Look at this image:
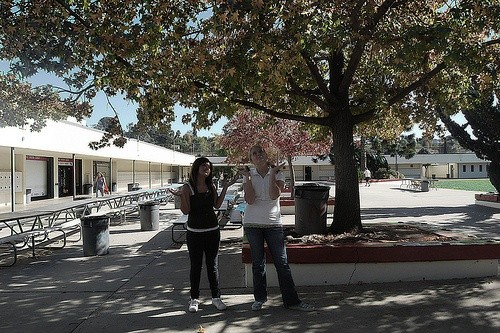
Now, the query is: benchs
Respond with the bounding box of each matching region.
[174,214,188,224]
[230,209,242,223]
[0,183,182,267]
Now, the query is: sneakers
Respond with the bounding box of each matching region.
[252,300,264,310]
[189,299,200,312]
[212,298,226,310]
[289,303,315,311]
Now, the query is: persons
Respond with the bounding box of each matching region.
[363,167,371,187]
[170,157,240,313]
[94,171,106,198]
[237,144,318,314]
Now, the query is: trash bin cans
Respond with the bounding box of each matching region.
[25,188,32,203]
[80,215,110,257]
[83,183,92,195]
[293,185,331,235]
[139,200,160,231]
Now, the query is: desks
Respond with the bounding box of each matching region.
[400,178,439,191]
[71,197,109,217]
[0,210,53,235]
[28,202,82,225]
[99,195,128,211]
[172,183,242,243]
